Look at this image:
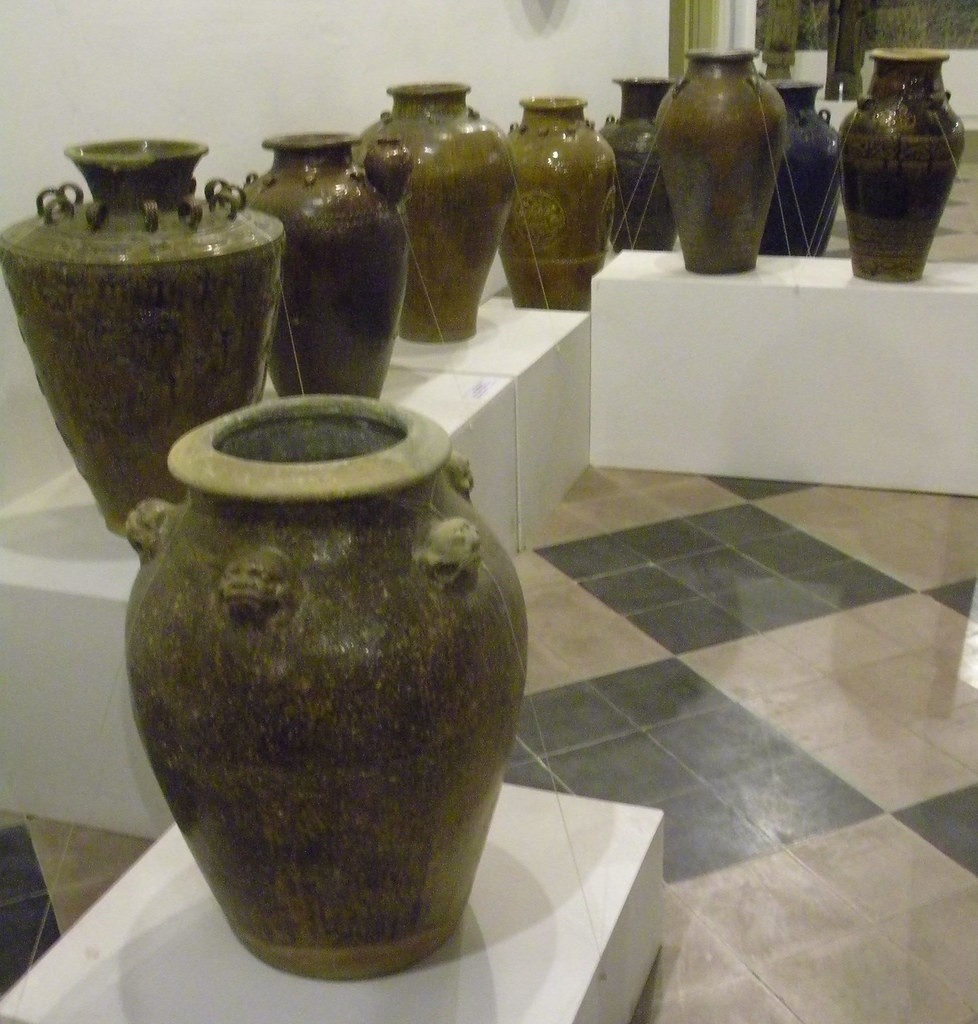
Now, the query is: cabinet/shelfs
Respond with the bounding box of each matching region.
[0,248,978,1023]
